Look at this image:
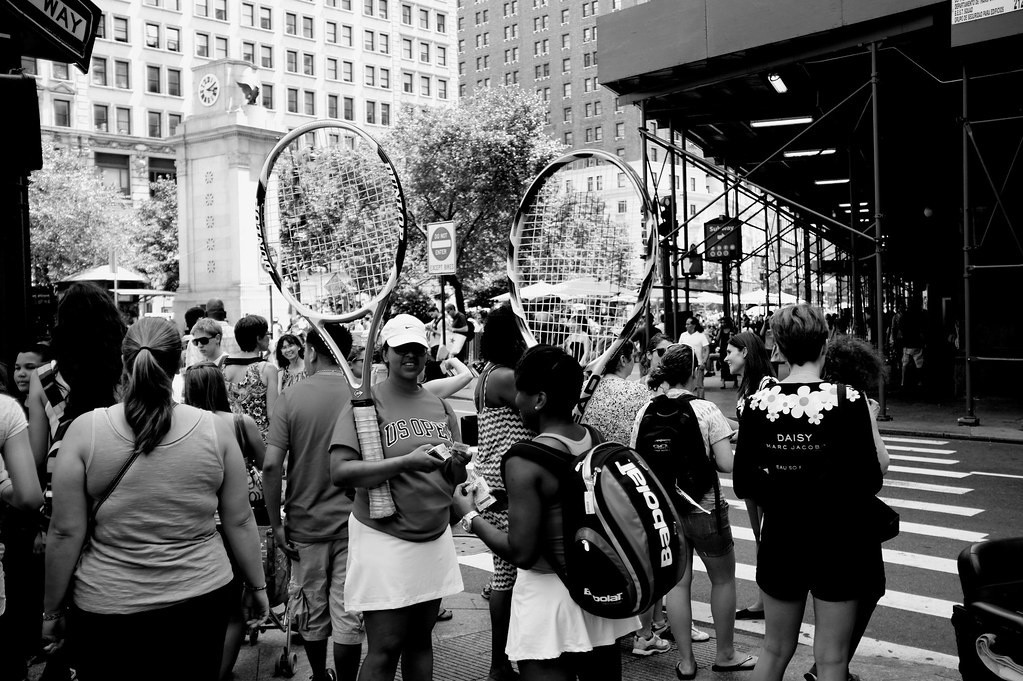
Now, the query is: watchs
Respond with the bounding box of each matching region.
[457,509,480,533]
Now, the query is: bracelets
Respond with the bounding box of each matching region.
[241,582,268,592]
[40,609,69,623]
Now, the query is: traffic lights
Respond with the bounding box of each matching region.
[657,196,676,236]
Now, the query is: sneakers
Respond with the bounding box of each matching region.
[632,620,710,655]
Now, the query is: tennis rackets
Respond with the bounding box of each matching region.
[502,146,661,425]
[252,117,412,521]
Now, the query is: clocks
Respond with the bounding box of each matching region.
[197,73,221,108]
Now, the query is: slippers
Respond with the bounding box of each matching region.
[675,659,697,680]
[712,655,758,672]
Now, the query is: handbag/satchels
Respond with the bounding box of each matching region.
[234,413,263,506]
[440,328,467,354]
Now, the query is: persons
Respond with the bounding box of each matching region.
[626,345,763,678]
[11,344,47,453]
[451,343,634,680]
[632,361,714,643]
[24,280,137,680]
[259,322,374,681]
[723,331,779,624]
[732,306,888,681]
[36,316,272,680]
[174,296,951,423]
[171,360,270,678]
[474,305,546,680]
[571,334,689,656]
[327,314,467,679]
[379,351,475,622]
[0,393,50,681]
[800,327,884,681]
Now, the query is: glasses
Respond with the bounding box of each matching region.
[447,310,451,313]
[393,345,426,356]
[666,344,694,379]
[191,337,216,346]
[265,332,273,339]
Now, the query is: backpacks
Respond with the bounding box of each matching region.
[465,321,475,342]
[500,421,688,618]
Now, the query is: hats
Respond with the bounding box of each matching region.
[380,313,429,349]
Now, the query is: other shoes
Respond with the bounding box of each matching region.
[735,607,764,619]
[804,665,860,681]
[437,608,452,620]
[309,668,336,681]
